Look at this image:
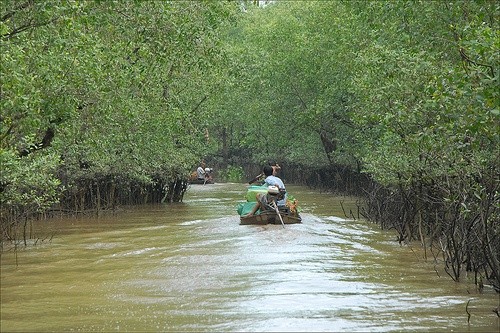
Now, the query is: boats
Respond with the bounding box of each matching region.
[239,202,303,226]
[188,172,215,185]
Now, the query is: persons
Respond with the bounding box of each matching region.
[197,164,205,180]
[247,191,275,217]
[263,165,286,207]
